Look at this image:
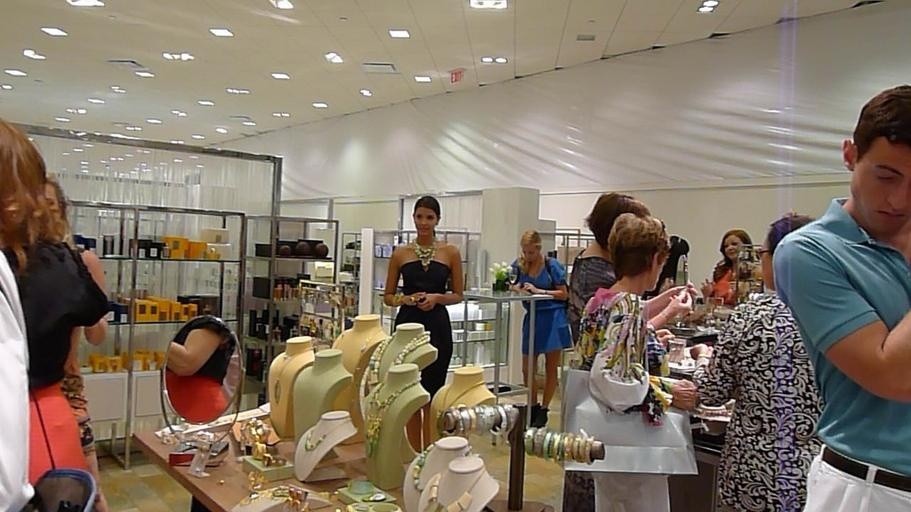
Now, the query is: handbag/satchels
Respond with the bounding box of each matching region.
[27,468,96,511]
[563,368,698,475]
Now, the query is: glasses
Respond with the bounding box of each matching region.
[757,249,770,259]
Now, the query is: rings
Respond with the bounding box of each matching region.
[410,296,417,302]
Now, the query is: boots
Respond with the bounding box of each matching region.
[531,402,548,428]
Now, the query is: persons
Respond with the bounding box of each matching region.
[0,119,112,511]
[687,213,833,511]
[775,83,911,511]
[701,227,756,304]
[383,195,466,408]
[504,229,574,430]
[0,250,39,512]
[164,315,237,425]
[42,176,109,511]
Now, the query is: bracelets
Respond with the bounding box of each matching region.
[660,311,672,323]
[437,403,595,465]
[393,292,405,307]
[545,289,548,294]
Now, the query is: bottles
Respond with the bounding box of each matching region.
[410,295,426,303]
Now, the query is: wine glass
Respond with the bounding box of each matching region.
[506,268,519,295]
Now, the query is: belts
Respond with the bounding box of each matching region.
[820,448,911,493]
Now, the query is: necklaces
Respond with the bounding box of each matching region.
[422,467,491,511]
[413,438,473,493]
[367,330,433,386]
[304,418,352,451]
[364,376,423,457]
[410,238,439,272]
[435,380,488,412]
[271,346,315,405]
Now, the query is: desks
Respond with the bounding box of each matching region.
[131,420,404,511]
